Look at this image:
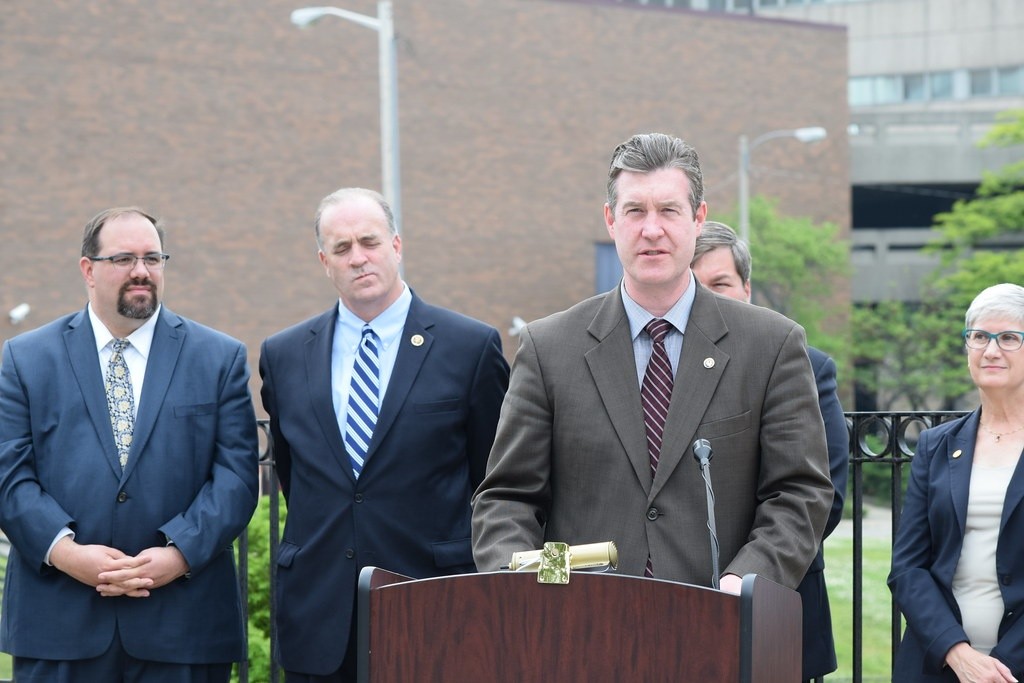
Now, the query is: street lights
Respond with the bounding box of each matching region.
[291,0,403,285]
[739,126,828,302]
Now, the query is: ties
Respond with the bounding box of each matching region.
[345,324,380,480]
[641,319,674,482]
[106,337,136,475]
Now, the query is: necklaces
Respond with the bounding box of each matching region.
[979,418,1024,442]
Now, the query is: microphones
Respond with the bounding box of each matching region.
[693,438,721,590]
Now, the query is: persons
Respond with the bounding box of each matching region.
[258,188,511,683]
[886,283,1024,683]
[690,221,851,683]
[0,206,259,683]
[470,133,835,594]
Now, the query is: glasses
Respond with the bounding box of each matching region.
[91,254,169,271]
[962,328,1024,351]
